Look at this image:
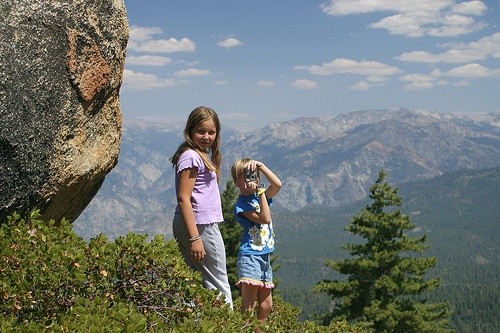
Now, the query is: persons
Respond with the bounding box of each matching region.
[168,106,235,314]
[231,157,282,333]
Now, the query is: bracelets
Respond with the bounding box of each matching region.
[257,188,266,197]
[189,235,202,241]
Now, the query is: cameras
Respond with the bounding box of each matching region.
[245,168,259,184]
